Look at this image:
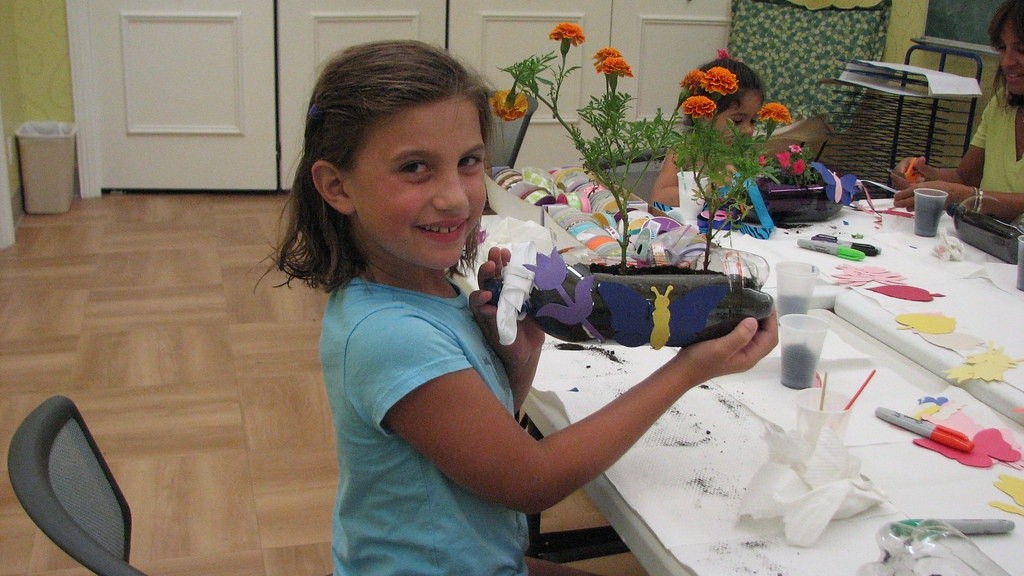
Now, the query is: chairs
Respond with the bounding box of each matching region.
[6,393,154,576]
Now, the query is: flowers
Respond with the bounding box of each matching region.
[756,143,822,187]
[484,18,792,273]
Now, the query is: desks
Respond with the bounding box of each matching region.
[444,192,1023,576]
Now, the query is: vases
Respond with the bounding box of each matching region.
[486,254,776,353]
[719,166,863,229]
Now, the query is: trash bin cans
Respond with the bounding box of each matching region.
[15,120,80,216]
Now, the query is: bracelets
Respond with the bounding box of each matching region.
[972,185,982,214]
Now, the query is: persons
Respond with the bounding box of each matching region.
[889,0,1024,229]
[650,57,767,208]
[257,38,778,576]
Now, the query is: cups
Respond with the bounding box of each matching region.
[1016,235,1024,291]
[914,188,949,238]
[795,387,853,450]
[677,171,708,221]
[779,314,829,390]
[774,260,819,325]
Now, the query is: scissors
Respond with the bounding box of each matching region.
[885,157,925,188]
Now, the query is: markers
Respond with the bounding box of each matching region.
[874,406,976,452]
[797,237,866,262]
[811,233,878,257]
[892,518,1016,536]
